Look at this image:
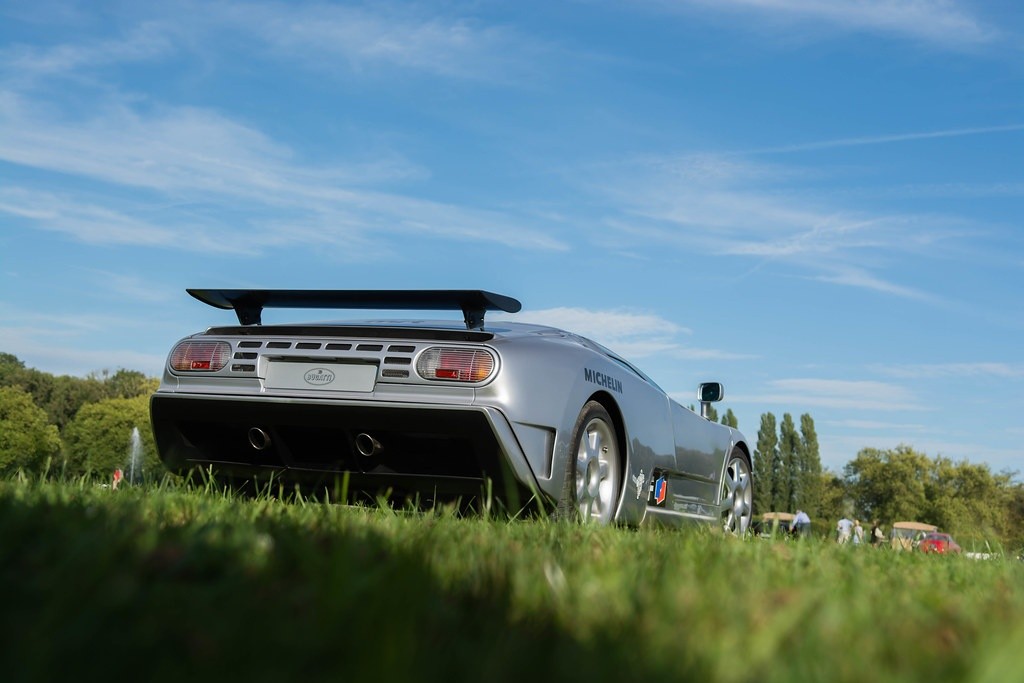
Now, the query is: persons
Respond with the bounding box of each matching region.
[789,509,811,534]
[869,520,877,544]
[112,467,123,490]
[836,515,854,543]
[874,522,887,544]
[853,520,863,542]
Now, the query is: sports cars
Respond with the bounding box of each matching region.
[150,287,756,535]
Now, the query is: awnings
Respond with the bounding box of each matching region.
[762,511,793,520]
[890,520,937,549]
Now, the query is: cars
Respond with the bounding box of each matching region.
[918,532,961,555]
